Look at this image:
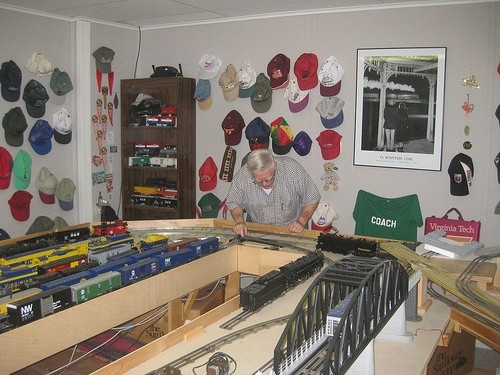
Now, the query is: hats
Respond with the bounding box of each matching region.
[447,152,472,196]
[198,156,218,191]
[1,51,76,240]
[217,50,346,160]
[198,193,222,219]
[311,200,337,231]
[93,45,115,72]
[196,51,223,80]
[193,79,213,110]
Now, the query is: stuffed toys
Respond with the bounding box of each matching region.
[321,163,341,191]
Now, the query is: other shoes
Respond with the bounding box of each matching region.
[386,147,395,152]
[397,147,404,152]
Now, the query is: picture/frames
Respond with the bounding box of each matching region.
[353,46,447,171]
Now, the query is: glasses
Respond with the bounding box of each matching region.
[251,167,276,184]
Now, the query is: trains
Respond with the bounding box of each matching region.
[326,283,381,337]
[240,249,325,311]
[1,219,219,325]
[316,233,377,258]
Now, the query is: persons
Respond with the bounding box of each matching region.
[224,148,321,237]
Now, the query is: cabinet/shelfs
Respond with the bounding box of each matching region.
[120,77,195,220]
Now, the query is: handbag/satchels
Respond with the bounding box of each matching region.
[424,207,480,244]
